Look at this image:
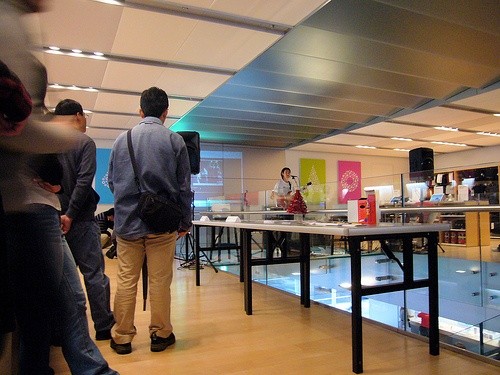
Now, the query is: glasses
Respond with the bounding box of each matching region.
[85,114,89,118]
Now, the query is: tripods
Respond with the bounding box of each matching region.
[176,232,218,274]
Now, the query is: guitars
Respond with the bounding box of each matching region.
[277,182,312,207]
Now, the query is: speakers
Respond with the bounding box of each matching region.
[177,131,200,174]
[409,148,434,183]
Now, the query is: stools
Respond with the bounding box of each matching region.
[210,204,239,261]
[327,216,347,254]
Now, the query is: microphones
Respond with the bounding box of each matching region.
[290,175,297,177]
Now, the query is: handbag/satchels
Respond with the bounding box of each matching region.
[137,190,185,234]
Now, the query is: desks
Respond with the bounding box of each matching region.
[192,220,452,374]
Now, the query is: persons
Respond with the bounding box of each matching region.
[0,50,116,375]
[472,183,485,197]
[423,190,432,200]
[270,168,294,207]
[107,86,193,354]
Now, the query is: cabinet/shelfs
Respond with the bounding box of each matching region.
[384,201,490,247]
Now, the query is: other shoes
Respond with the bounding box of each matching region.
[150,333,176,352]
[95,326,137,340]
[109,338,133,355]
